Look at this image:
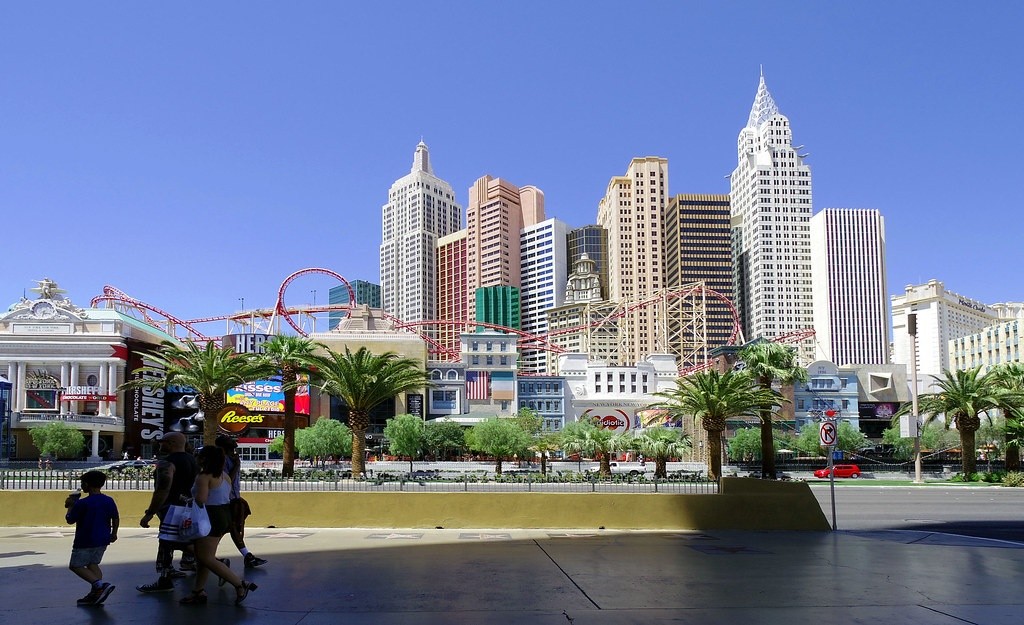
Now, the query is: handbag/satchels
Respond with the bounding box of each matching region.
[230,496,251,531]
[157,496,190,542]
[178,499,212,540]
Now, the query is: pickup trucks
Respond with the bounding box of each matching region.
[590,462,649,478]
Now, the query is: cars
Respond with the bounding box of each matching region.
[814,464,861,479]
[106,459,157,477]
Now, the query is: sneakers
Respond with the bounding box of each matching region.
[136,578,174,593]
[217,558,230,586]
[77,592,94,605]
[90,582,116,606]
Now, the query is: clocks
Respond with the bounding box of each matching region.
[31,302,54,318]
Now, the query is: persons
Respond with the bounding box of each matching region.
[64,470,119,605]
[136,432,267,592]
[179,446,257,604]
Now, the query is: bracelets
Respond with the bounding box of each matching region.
[145,510,151,516]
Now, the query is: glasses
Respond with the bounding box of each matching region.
[158,439,175,444]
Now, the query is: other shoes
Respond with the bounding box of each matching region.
[168,569,188,578]
[242,552,268,568]
[182,562,197,572]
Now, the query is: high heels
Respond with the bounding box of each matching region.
[179,588,207,605]
[234,580,258,604]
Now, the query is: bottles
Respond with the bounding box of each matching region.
[64,488,82,508]
